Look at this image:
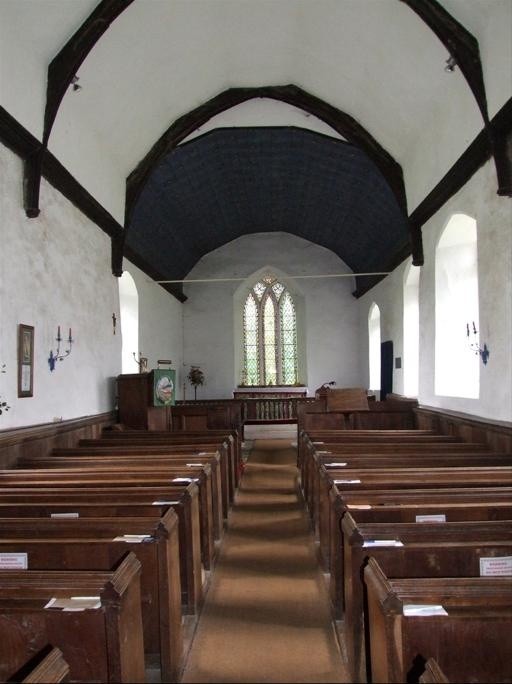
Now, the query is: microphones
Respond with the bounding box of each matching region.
[322,382,335,387]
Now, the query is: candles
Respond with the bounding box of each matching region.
[467,323,469,333]
[472,321,476,331]
[58,326,60,338]
[69,328,71,337]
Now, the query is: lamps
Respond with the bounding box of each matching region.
[445,57,457,72]
[70,74,82,93]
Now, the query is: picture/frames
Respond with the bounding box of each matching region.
[18,324,34,398]
